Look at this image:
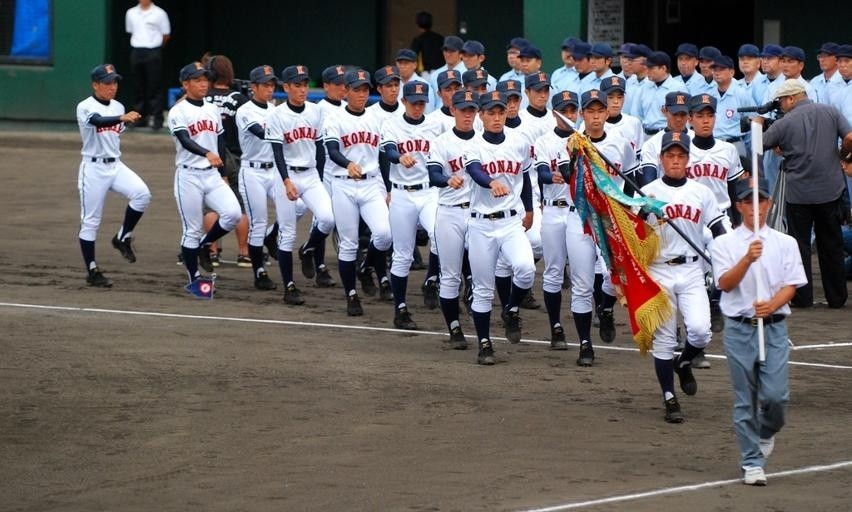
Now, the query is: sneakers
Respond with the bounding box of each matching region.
[448,319,467,349]
[597,305,616,343]
[577,339,594,367]
[86,266,113,288]
[551,323,568,350]
[237,223,424,317]
[111,232,136,263]
[477,338,495,365]
[394,303,417,331]
[707,288,724,332]
[198,235,220,272]
[672,326,711,369]
[673,354,697,396]
[421,274,439,310]
[759,434,774,458]
[741,462,767,486]
[501,293,541,344]
[664,391,683,423]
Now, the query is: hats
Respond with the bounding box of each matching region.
[689,94,718,113]
[91,63,122,83]
[736,176,770,200]
[617,43,852,70]
[661,131,690,153]
[437,35,626,111]
[771,79,806,104]
[665,90,691,115]
[178,49,430,103]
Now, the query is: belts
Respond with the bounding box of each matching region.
[666,255,699,265]
[290,166,309,174]
[544,199,576,212]
[394,182,435,192]
[334,173,376,181]
[250,162,274,170]
[184,165,213,171]
[91,157,115,163]
[453,201,470,209]
[727,313,785,327]
[471,209,517,221]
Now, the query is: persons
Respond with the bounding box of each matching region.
[628,132,728,424]
[125,0,173,130]
[76,63,152,289]
[379,36,546,365]
[706,174,808,489]
[410,10,447,77]
[269,62,402,315]
[167,56,308,298]
[534,36,852,367]
